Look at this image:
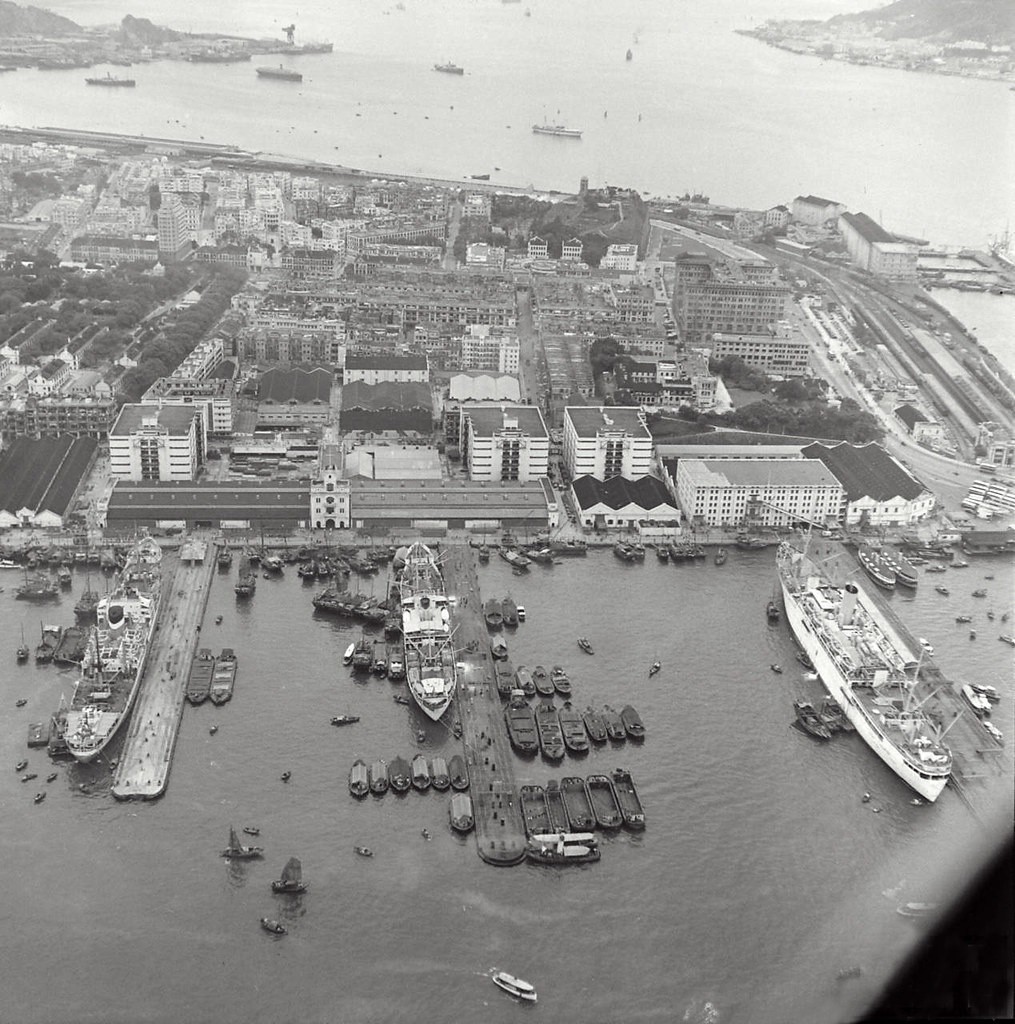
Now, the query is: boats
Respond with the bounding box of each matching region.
[1,517,1015,934]
[773,523,967,801]
[389,540,459,722]
[55,519,165,765]
[492,970,539,1004]
